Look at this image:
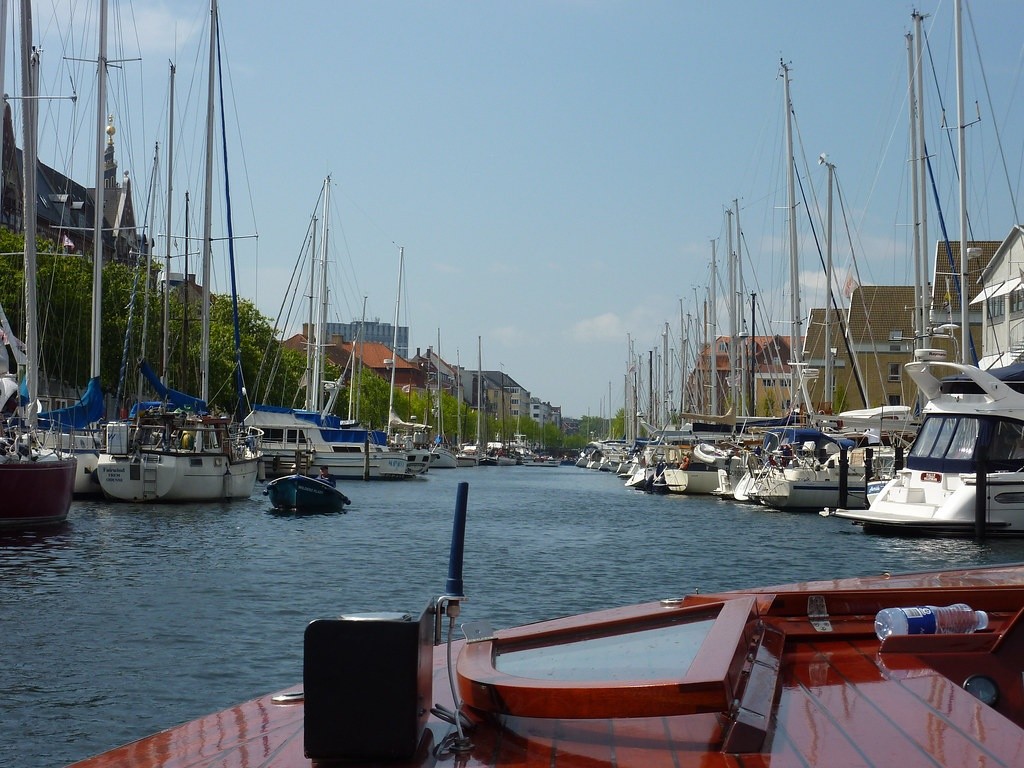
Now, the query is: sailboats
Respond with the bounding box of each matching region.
[3,1,1023,543]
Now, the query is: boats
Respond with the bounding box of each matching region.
[59,480,1024,768]
[264,477,353,516]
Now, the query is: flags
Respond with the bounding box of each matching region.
[841,264,859,299]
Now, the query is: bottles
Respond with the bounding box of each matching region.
[874,603,990,640]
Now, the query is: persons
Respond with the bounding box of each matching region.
[316,465,336,489]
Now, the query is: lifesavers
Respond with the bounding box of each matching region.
[681,456,690,469]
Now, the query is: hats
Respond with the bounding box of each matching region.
[320,465,328,469]
